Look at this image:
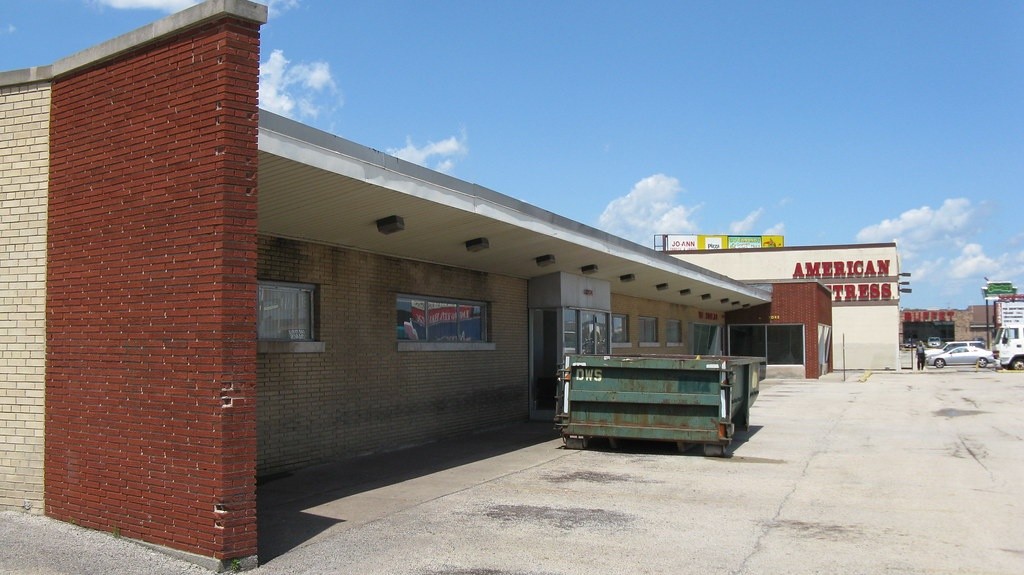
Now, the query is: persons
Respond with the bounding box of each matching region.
[916,341,926,370]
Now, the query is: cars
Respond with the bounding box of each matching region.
[928,337,941,348]
[926,346,994,368]
[904,341,916,348]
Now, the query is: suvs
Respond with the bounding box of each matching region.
[922,340,985,365]
[992,323,1024,370]
